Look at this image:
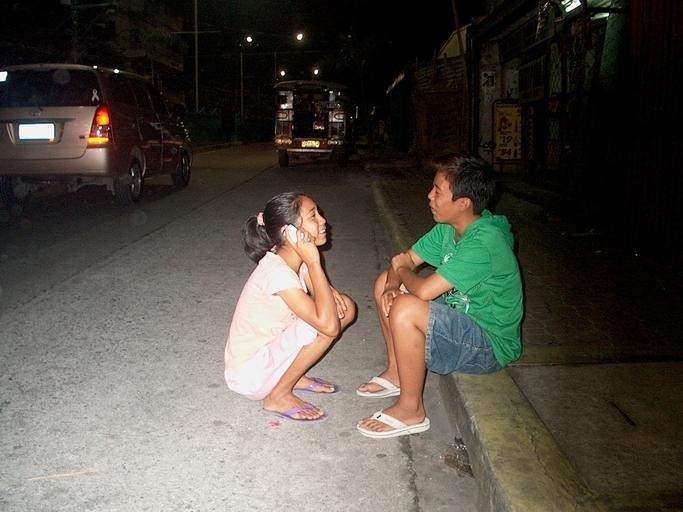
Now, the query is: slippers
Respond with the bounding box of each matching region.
[356,407,431,438]
[262,395,330,424]
[356,377,401,398]
[293,377,342,396]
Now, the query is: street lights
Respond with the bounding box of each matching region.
[239,32,254,118]
[271,25,323,81]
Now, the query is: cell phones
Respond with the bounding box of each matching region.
[282,223,310,244]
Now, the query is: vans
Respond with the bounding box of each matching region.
[0,61,196,204]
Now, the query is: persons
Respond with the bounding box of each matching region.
[355,152,523,439]
[221,193,359,423]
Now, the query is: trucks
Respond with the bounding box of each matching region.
[268,80,354,170]
